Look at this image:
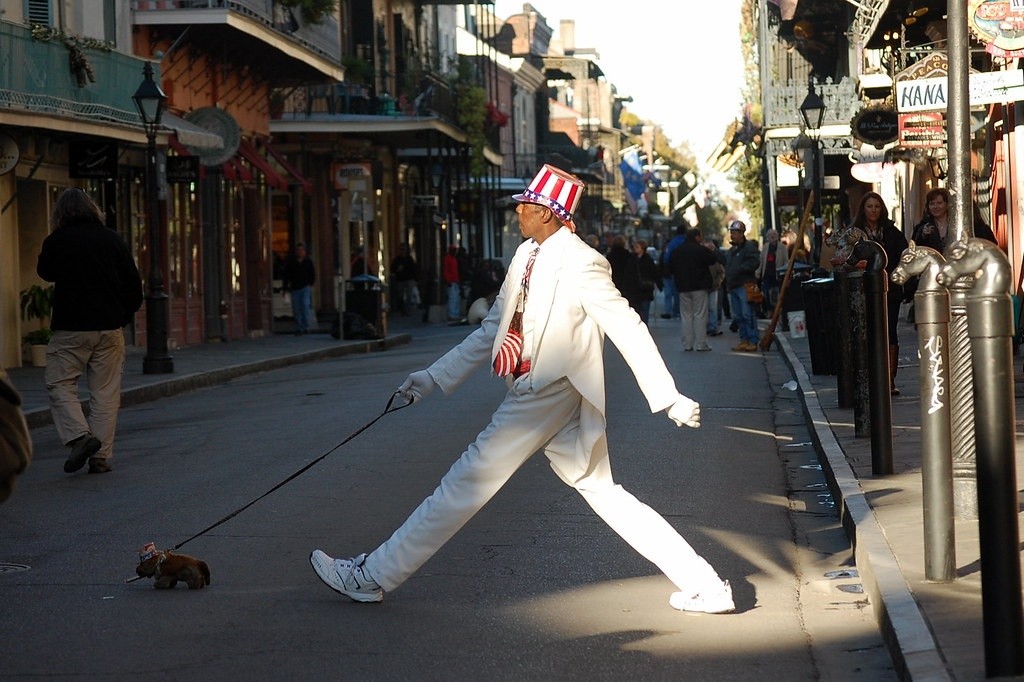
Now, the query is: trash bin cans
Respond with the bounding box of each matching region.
[344,274,384,340]
[776,261,812,331]
[800,277,839,376]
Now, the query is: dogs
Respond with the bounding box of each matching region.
[136,542,210,589]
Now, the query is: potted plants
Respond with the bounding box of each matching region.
[18,284,55,368]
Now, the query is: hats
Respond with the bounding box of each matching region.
[449,243,460,250]
[511,164,586,233]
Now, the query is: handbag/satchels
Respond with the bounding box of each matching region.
[746,283,763,304]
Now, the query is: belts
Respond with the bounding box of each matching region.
[511,360,530,379]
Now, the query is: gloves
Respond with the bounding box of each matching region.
[396,370,437,408]
[666,393,701,428]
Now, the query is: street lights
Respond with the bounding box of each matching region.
[131,60,178,374]
[798,76,828,278]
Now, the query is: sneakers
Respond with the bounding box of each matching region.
[669,579,735,614]
[310,550,383,602]
[88,458,112,473]
[64,435,102,473]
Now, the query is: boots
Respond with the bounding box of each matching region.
[889,344,900,395]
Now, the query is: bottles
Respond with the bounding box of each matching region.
[745,283,761,302]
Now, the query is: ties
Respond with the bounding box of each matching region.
[492,247,541,378]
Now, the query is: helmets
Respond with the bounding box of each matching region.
[728,221,746,231]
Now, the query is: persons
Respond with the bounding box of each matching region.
[578,225,789,355]
[908,189,999,328]
[443,245,481,321]
[390,242,421,316]
[280,242,316,334]
[0,364,34,507]
[35,189,145,474]
[309,165,735,615]
[846,193,912,395]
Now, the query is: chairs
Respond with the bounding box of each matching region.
[307,79,375,115]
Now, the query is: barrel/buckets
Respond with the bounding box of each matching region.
[787,311,806,338]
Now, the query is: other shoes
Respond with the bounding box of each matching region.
[731,341,757,351]
[712,330,722,336]
[697,344,712,351]
[685,344,693,351]
[729,324,737,332]
[661,313,670,318]
